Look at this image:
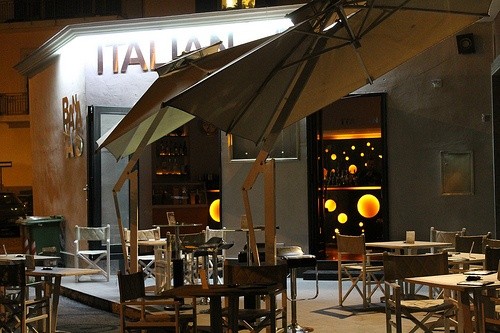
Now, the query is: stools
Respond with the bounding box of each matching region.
[239,242,265,324]
[278,244,319,333]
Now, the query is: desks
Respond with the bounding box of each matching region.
[404,274,500,333]
[25,267,101,333]
[161,283,287,333]
[209,226,279,265]
[0,253,61,300]
[153,224,203,259]
[365,240,452,300]
[428,252,485,270]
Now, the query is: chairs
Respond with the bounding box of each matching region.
[117,227,288,333]
[335,226,500,333]
[0,222,112,333]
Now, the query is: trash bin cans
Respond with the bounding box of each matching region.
[16,214,65,267]
